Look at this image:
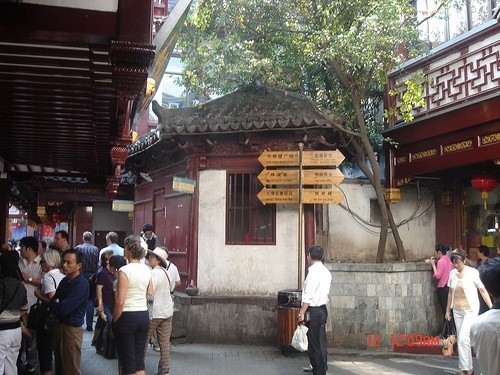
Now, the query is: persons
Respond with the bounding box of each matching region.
[0,230,129,375]
[297,246,332,375]
[146,246,177,375]
[430,242,500,375]
[112,235,154,375]
[0,252,29,375]
[140,223,160,261]
[48,248,91,375]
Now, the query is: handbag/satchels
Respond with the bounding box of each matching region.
[291,321,309,352]
[442,339,452,356]
[26,298,52,331]
[103,325,119,359]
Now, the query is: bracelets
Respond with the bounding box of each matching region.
[28,278,33,283]
[298,313,304,317]
[100,309,104,312]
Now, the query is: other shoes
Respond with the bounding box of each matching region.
[151,346,160,351]
[303,365,328,372]
[25,363,37,375]
[86,327,93,331]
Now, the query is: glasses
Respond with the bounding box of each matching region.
[40,260,45,264]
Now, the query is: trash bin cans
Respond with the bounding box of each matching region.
[276,289,306,356]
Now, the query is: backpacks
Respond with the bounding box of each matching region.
[88,271,95,300]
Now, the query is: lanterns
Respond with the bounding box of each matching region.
[471,173,497,211]
[17,221,22,228]
[53,210,66,224]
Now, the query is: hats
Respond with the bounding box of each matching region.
[147,247,170,269]
